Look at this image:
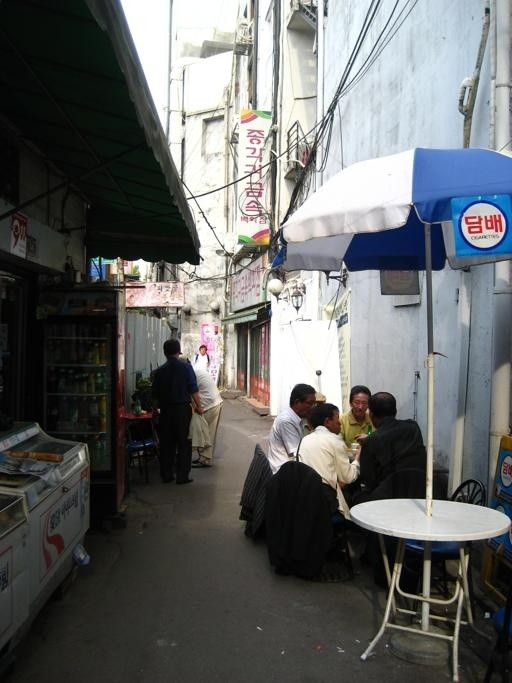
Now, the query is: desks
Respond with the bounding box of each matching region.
[348,497,512,683]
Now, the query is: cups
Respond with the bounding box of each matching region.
[351,442,361,448]
[73,270,81,282]
[352,449,357,458]
[88,275,98,282]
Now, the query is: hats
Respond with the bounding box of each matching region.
[163,339,183,355]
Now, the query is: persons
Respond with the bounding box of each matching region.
[148,338,224,482]
[267,382,428,574]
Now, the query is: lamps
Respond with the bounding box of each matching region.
[290,279,307,312]
[266,277,289,304]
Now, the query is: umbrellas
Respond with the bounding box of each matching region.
[274,148,512,517]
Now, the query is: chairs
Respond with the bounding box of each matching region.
[125,420,156,483]
[242,443,274,547]
[403,478,487,625]
[283,461,357,582]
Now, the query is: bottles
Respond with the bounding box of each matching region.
[70,435,107,469]
[65,255,74,281]
[51,396,106,432]
[367,423,373,435]
[50,367,108,392]
[134,394,142,414]
[48,339,107,364]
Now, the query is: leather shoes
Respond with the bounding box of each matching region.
[163,458,211,484]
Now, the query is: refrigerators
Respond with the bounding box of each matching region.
[40,282,128,514]
